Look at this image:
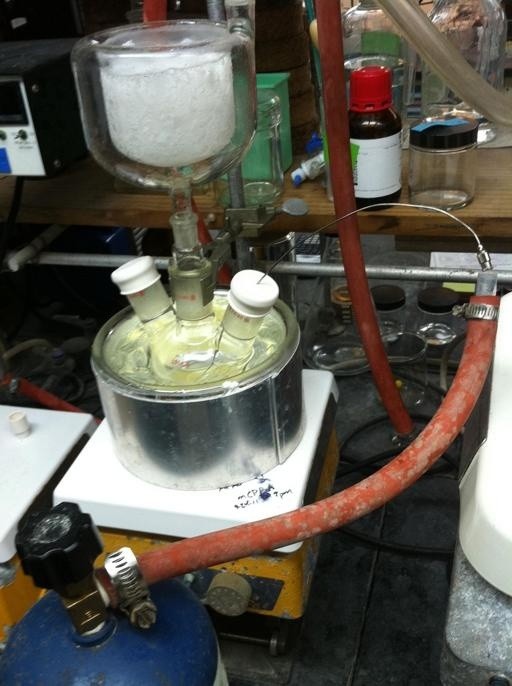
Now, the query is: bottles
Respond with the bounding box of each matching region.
[370,285,407,342]
[414,286,462,345]
[407,117,477,213]
[347,65,405,212]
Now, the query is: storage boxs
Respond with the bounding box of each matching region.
[219,70,293,180]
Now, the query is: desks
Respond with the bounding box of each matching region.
[0,144,511,291]
